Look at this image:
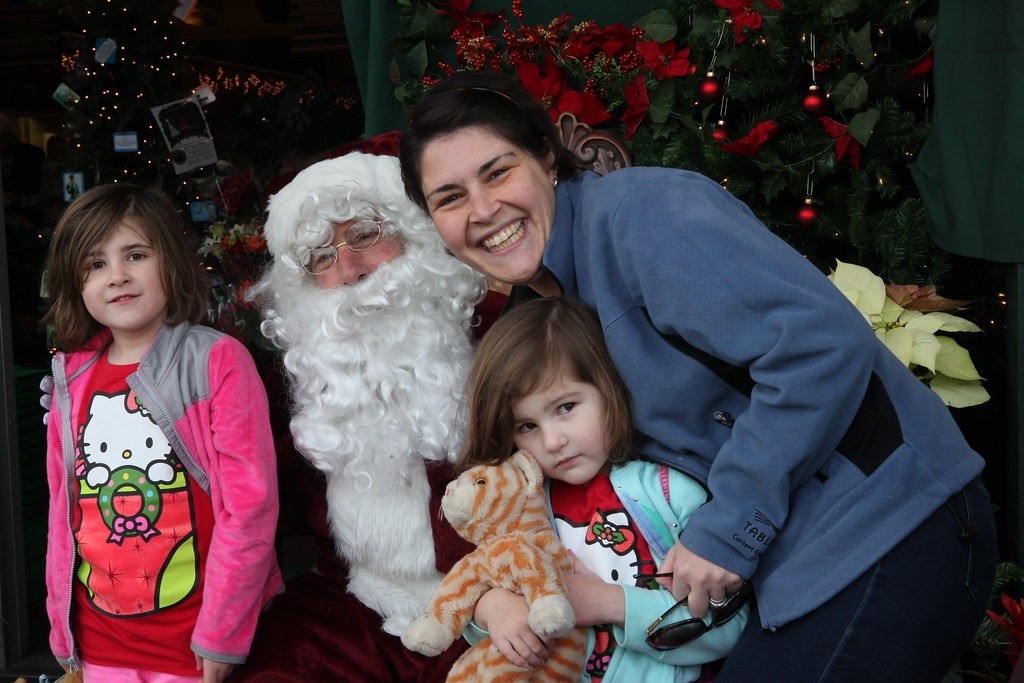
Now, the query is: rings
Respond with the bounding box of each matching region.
[710,598,725,607]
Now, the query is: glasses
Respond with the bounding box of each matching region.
[633,570,750,652]
[301,217,390,274]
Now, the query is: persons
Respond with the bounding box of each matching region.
[393,72,1004,683]
[456,296,748,683]
[39,131,508,683]
[37,181,283,683]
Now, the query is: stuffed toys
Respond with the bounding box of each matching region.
[401,446,589,683]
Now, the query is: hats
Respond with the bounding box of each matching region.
[265,132,412,261]
